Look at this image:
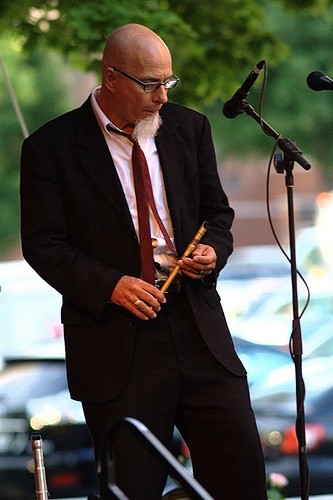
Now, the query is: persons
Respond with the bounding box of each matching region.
[21,24,268,500]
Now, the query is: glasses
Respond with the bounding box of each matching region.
[111,66,179,93]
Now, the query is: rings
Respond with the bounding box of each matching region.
[135,300,141,304]
[200,270,204,275]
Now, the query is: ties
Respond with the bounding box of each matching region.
[107,125,174,286]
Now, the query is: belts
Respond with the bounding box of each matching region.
[155,279,183,294]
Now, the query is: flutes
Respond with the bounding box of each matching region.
[143,219,210,318]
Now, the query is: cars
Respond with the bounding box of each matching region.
[0,244,333,500]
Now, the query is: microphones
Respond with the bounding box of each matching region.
[306,71,333,91]
[222,60,265,118]
